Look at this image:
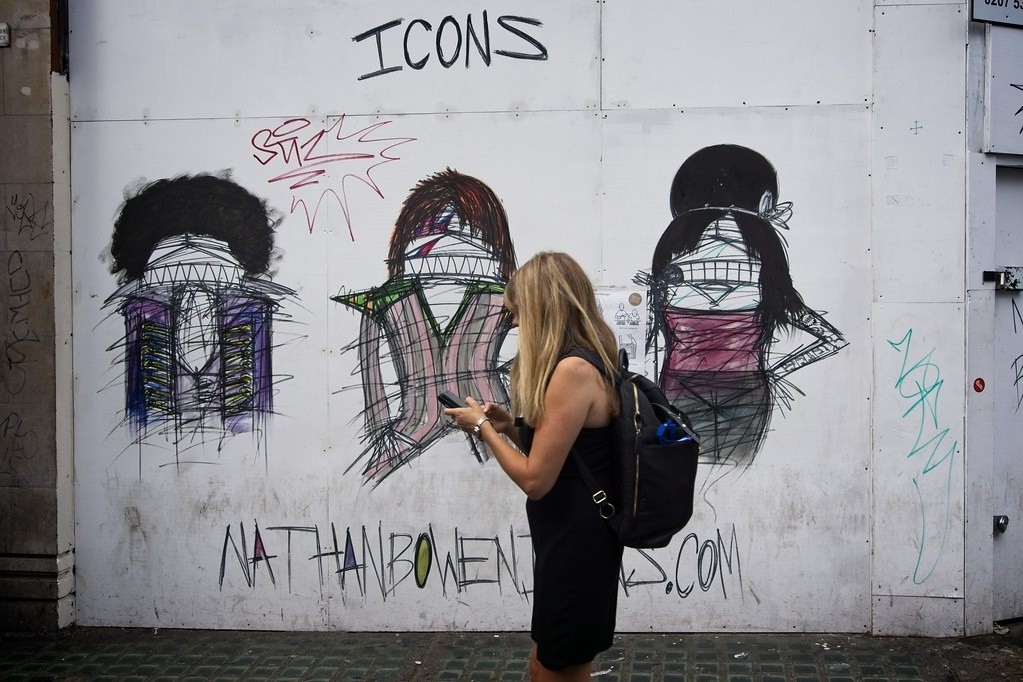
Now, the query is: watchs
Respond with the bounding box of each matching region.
[473,418,493,441]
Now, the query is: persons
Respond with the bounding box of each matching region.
[446,252,621,682]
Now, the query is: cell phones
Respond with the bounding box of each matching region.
[438,391,469,410]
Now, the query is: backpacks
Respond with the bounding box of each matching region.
[542,345,704,550]
